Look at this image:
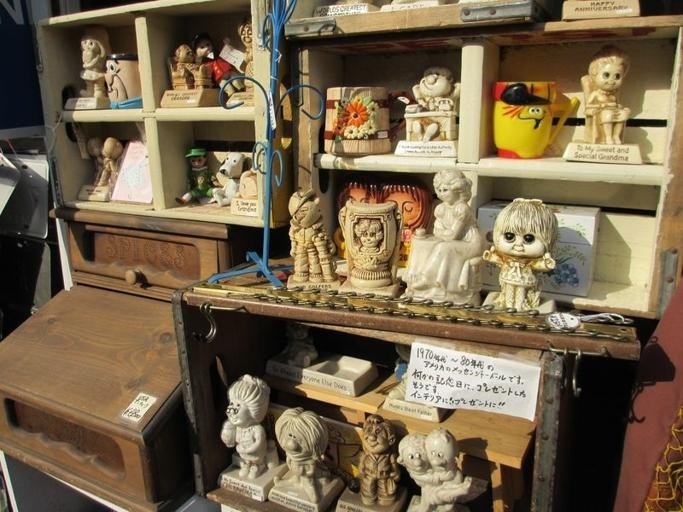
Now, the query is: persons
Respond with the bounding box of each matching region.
[76,30,109,100]
[267,406,343,511]
[190,31,244,99]
[395,429,474,511]
[216,373,288,502]
[422,428,464,511]
[335,413,410,511]
[581,43,633,148]
[174,146,213,203]
[234,16,254,94]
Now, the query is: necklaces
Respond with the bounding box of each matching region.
[597,89,617,96]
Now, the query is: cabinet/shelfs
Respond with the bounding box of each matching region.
[284,15,683,319]
[0,208,257,512]
[36,0,333,230]
[172,256,641,512]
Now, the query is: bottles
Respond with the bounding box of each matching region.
[104,51,142,110]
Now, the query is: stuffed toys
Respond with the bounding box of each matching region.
[212,151,250,206]
[96,137,123,187]
[87,135,103,185]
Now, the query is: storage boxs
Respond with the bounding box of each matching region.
[477,200,601,297]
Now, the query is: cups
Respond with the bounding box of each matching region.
[489,80,580,161]
